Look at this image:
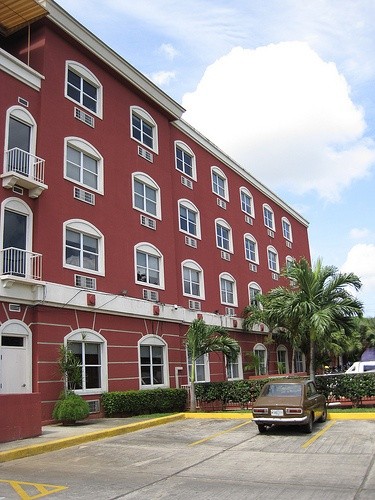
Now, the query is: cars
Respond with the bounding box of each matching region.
[251,379,327,433]
[345,361,375,374]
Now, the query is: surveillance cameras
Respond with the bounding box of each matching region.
[174,304,178,310]
